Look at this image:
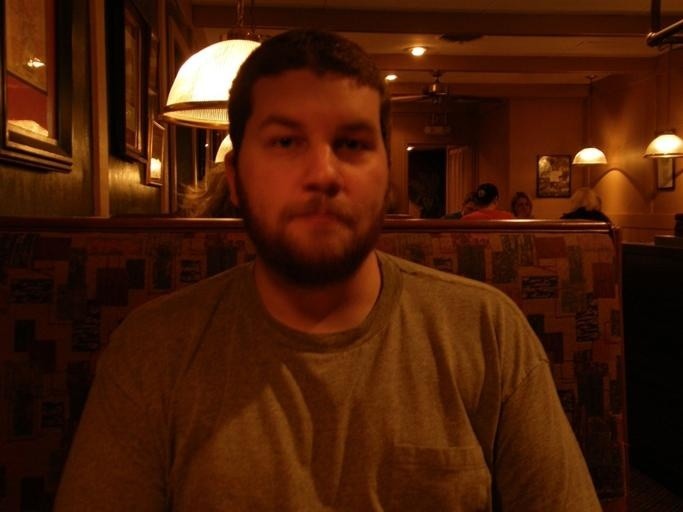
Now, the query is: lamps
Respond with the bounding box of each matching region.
[641,128,683,160]
[160,38,263,131]
[571,147,608,166]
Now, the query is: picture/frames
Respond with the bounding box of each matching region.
[107,3,166,186]
[0,0,76,172]
[654,128,676,192]
[537,153,572,199]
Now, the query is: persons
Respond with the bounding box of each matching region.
[511,191,534,218]
[459,181,514,221]
[440,191,478,220]
[559,188,612,224]
[188,163,240,218]
[51,29,602,511]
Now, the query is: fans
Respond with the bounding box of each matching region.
[388,67,504,106]
